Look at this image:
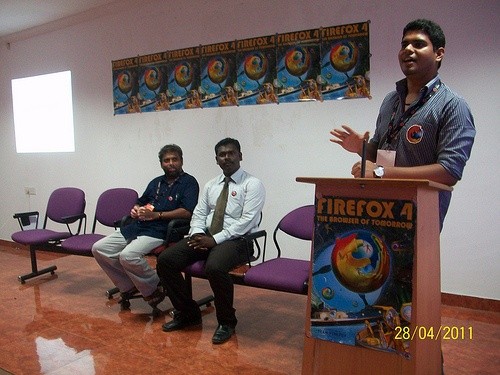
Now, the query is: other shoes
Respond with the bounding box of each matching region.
[148,287,166,305]
[118,286,144,302]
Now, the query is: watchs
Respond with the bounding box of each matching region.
[373,165,384,178]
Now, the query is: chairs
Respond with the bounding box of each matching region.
[11,187,315,318]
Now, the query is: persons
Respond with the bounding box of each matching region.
[155,137,264,344]
[329,18,476,375]
[91,143,200,306]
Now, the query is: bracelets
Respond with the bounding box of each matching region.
[159,211,163,218]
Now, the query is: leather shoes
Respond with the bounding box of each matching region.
[212,324,234,345]
[163,307,202,332]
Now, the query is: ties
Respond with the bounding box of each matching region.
[209,176,232,236]
